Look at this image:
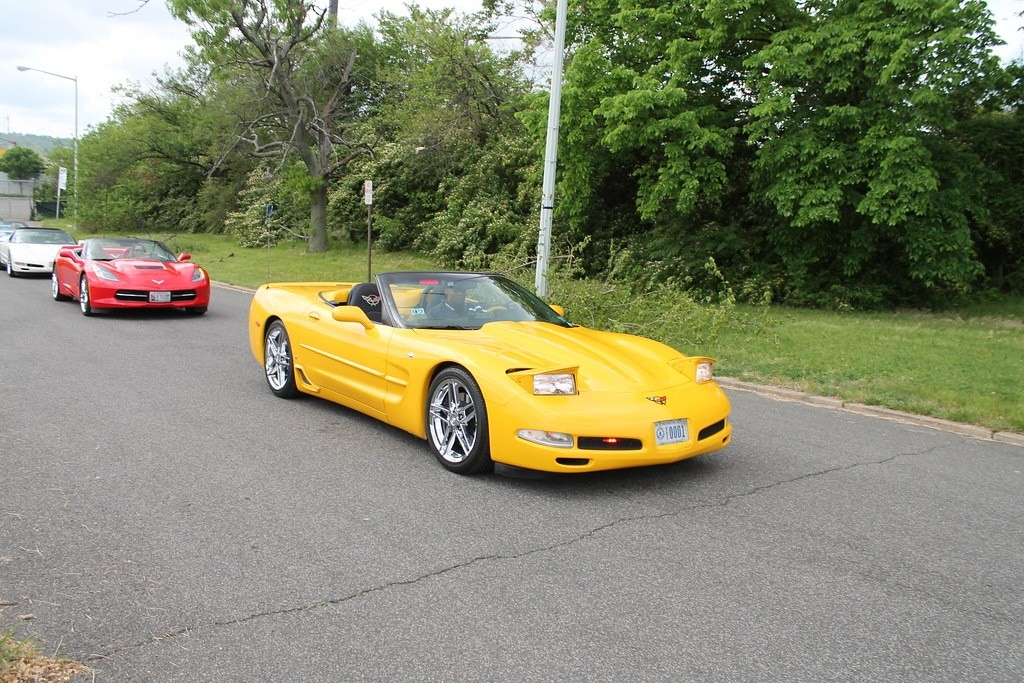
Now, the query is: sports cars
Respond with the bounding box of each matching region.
[49,233,211,318]
[247,271,731,476]
[1,227,79,278]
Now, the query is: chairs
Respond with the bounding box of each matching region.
[78,245,107,259]
[346,282,383,323]
[124,247,134,258]
[46,233,61,244]
[20,235,33,243]
[420,284,448,319]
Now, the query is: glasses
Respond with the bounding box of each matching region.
[135,249,142,252]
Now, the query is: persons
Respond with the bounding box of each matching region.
[128,243,151,258]
[47,232,58,241]
[427,286,494,317]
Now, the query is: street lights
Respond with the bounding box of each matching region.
[18,65,79,234]
